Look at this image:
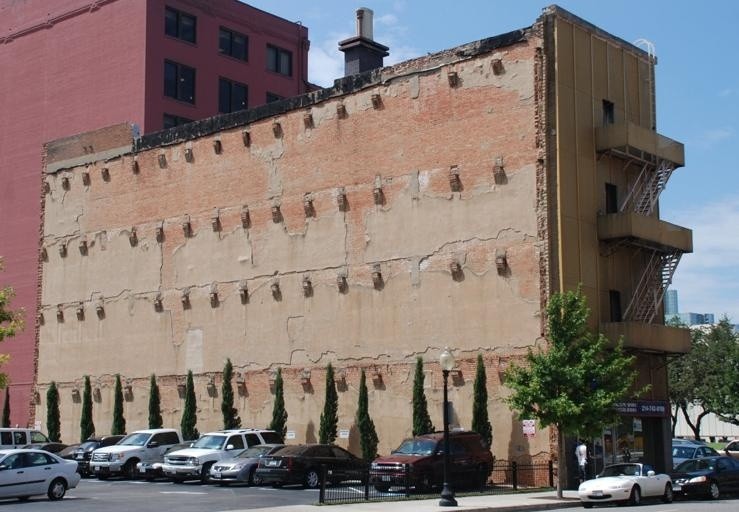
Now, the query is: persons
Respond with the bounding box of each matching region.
[575,438,589,483]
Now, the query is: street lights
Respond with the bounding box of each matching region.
[437,346,460,506]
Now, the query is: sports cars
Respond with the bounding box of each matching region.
[578,462,673,507]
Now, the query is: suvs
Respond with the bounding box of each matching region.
[162,425,285,485]
[369,429,494,497]
[89,427,183,480]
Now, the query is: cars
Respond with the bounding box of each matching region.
[209,444,287,487]
[1,448,82,502]
[607,447,646,465]
[671,437,738,501]
[255,444,364,489]
[135,440,196,482]
[22,433,128,479]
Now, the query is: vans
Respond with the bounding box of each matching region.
[0,427,51,459]
